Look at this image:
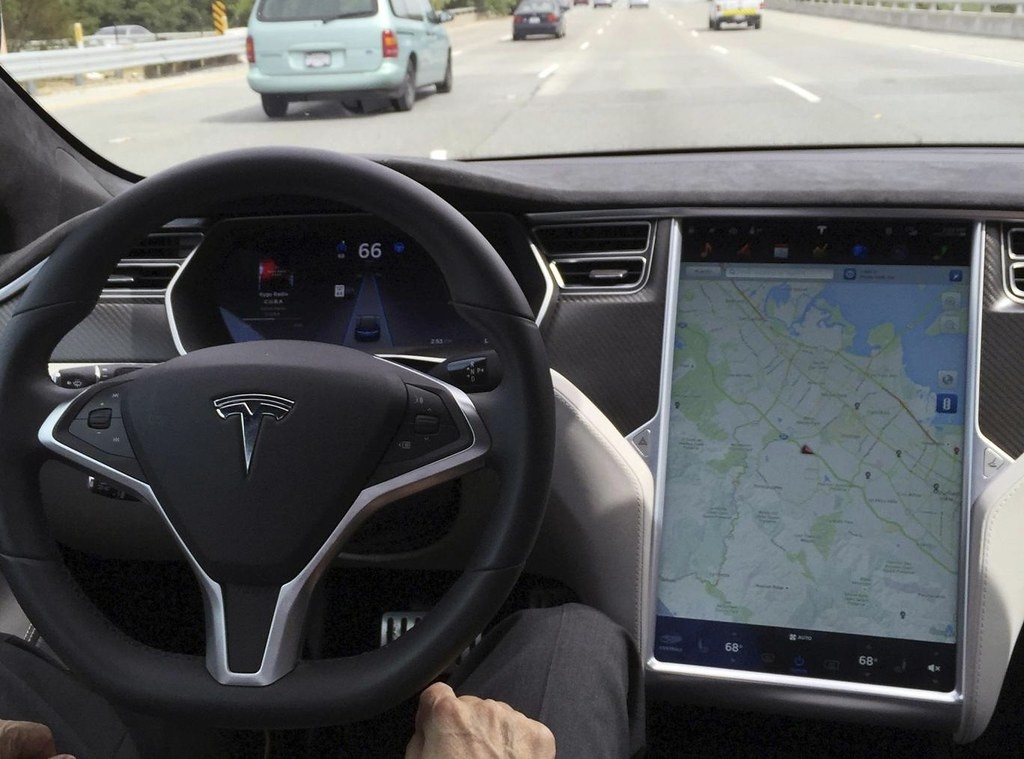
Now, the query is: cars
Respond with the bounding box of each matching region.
[594,0,612,7]
[708,0,763,31]
[511,0,569,41]
[246,0,454,118]
[630,0,649,9]
[574,0,588,6]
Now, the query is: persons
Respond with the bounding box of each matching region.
[0,602,648,759]
